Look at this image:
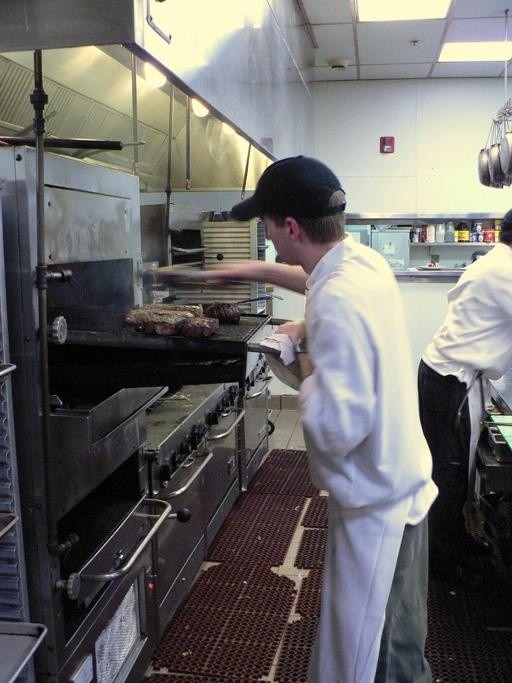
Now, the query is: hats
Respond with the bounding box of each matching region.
[231,155,346,221]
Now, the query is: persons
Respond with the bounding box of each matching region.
[416,206,512,586]
[153,149,440,683]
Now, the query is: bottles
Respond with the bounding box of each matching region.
[412,221,471,243]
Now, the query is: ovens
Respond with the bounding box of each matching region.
[2,183,276,527]
[22,319,309,682]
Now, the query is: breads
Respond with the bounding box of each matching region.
[137,303,204,317]
[183,316,220,339]
[205,301,240,325]
[124,308,193,336]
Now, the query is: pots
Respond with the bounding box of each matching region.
[477,113,512,189]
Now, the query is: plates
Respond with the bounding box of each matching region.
[421,264,466,270]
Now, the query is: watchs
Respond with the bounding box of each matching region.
[291,336,310,353]
